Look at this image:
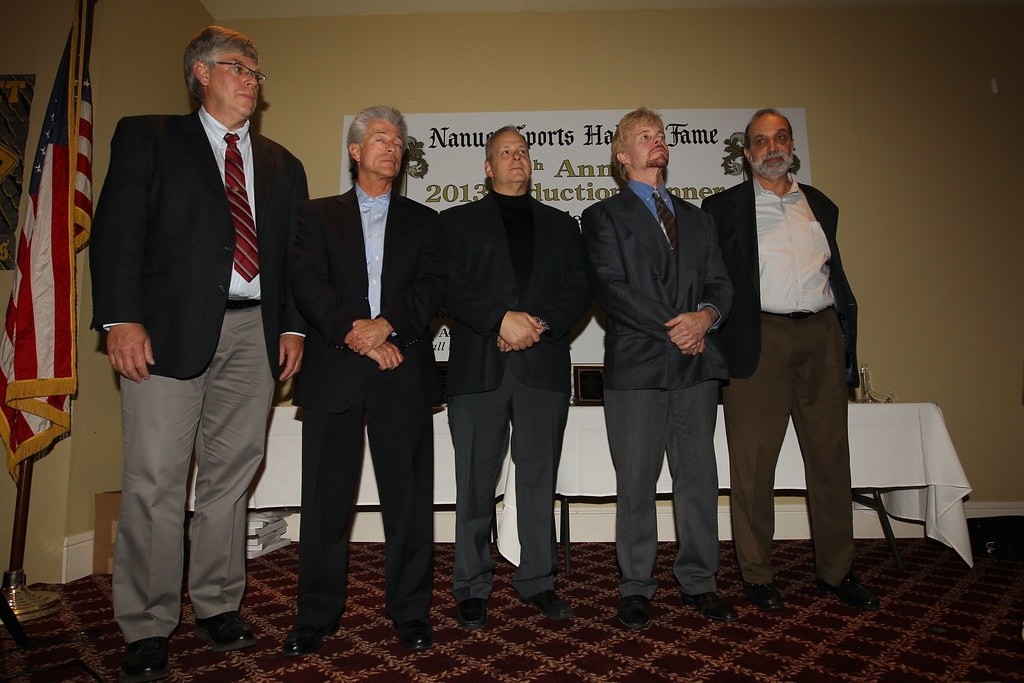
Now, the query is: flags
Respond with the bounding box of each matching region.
[0,0,99,483]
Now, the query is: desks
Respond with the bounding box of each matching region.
[183,392,975,579]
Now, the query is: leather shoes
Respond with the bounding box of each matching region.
[819,572,877,607]
[617,594,652,629]
[398,616,434,649]
[195,611,258,652]
[119,636,171,683]
[679,589,739,620]
[457,598,488,630]
[283,623,336,655]
[529,589,573,620]
[745,580,785,614]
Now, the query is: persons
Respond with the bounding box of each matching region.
[700,109,881,611]
[283,105,446,654]
[437,125,594,627]
[90,26,309,683]
[581,107,736,627]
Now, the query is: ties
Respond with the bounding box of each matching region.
[652,189,678,266]
[223,132,260,283]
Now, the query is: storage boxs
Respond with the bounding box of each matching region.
[91,489,123,574]
[244,518,291,559]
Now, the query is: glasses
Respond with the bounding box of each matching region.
[215,61,266,85]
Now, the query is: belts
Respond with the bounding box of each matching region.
[768,305,831,319]
[225,299,262,312]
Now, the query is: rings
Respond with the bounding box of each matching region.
[355,346,361,351]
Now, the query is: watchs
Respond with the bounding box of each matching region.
[533,316,551,332]
